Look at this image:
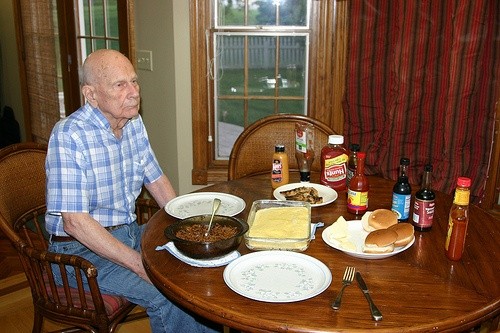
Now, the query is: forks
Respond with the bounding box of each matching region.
[331,266,356,310]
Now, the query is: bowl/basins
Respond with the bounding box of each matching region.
[163,213,249,258]
[245,199,312,251]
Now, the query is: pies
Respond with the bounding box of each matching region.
[280,186,322,204]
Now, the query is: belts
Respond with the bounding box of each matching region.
[49,224,116,242]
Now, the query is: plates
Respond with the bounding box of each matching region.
[223,250,333,304]
[273,182,338,208]
[322,220,416,259]
[164,192,247,221]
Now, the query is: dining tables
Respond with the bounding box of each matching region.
[141,169,500,333]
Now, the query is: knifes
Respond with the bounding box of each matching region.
[355,271,383,319]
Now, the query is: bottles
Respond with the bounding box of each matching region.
[391,157,412,223]
[270,144,289,189]
[319,135,352,191]
[346,145,363,185]
[412,164,436,232]
[293,121,316,181]
[347,151,369,215]
[445,177,471,261]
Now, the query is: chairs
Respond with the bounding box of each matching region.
[0,139,165,332]
[227,112,348,186]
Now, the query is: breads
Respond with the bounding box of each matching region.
[327,209,414,254]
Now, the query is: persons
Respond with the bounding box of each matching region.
[45,49,223,333]
